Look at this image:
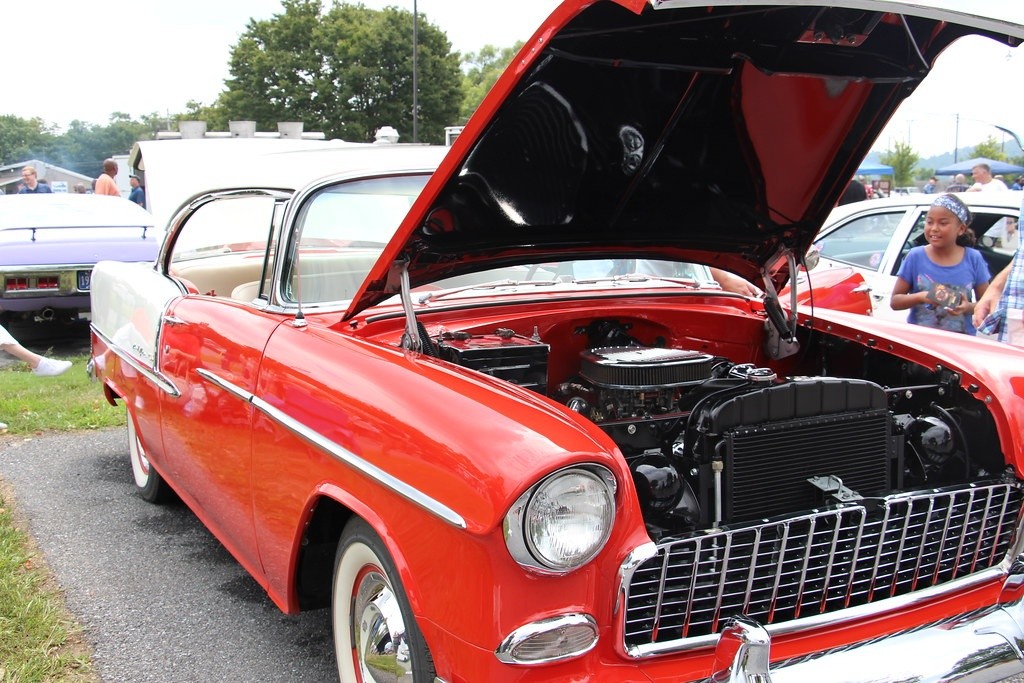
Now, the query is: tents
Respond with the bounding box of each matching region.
[856,162,895,192]
[935,158,1024,175]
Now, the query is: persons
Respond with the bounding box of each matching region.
[17,179,48,190]
[95,158,122,197]
[972,184,1024,346]
[946,173,971,192]
[77,179,97,193]
[965,164,1009,246]
[890,194,992,337]
[994,175,1024,190]
[838,175,885,206]
[17,166,53,194]
[1002,217,1020,250]
[0,322,73,432]
[129,175,144,207]
[923,177,939,194]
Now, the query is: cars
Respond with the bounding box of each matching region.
[89,0,1024,683]
[0,192,162,332]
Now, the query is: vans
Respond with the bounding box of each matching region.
[798,195,1024,323]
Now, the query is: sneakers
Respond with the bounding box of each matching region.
[31,355,72,377]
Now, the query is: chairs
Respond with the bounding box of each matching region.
[232,270,370,303]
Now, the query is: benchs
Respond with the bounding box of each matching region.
[178,254,378,298]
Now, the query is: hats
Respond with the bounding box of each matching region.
[129,175,141,183]
[931,177,939,181]
